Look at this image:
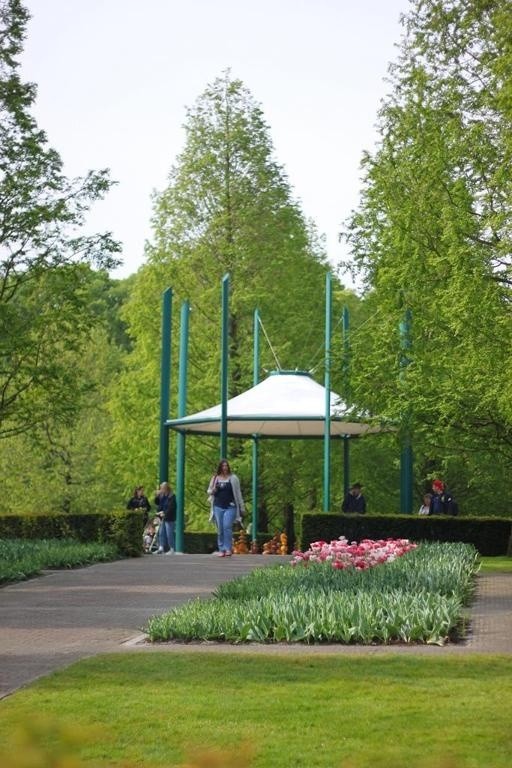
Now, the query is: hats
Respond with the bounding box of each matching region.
[349,482,363,490]
[424,493,433,498]
[433,480,445,490]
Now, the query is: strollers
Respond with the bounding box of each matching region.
[140,513,167,554]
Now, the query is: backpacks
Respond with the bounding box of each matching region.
[444,498,459,516]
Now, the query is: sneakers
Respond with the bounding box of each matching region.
[152,546,164,555]
[225,550,232,556]
[216,552,225,557]
[165,546,174,556]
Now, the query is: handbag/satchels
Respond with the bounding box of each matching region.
[207,494,212,503]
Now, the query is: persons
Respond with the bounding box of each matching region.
[341,481,366,513]
[205,459,248,556]
[126,484,151,529]
[153,481,177,555]
[429,479,454,515]
[417,492,431,515]
